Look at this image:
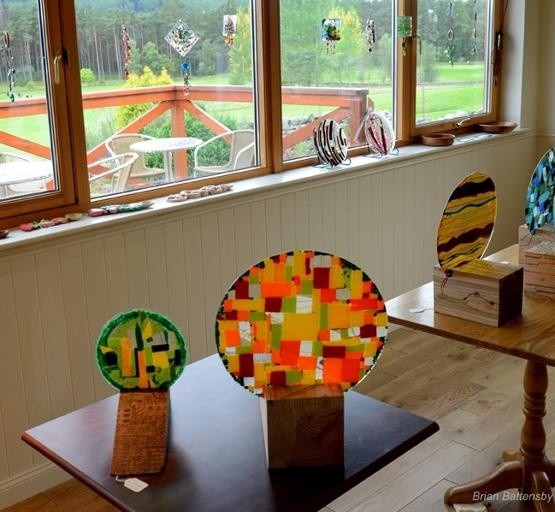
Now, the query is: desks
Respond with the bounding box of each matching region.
[20,236,555,512]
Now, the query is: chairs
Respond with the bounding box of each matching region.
[0,129,259,200]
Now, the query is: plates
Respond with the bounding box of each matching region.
[478,121,517,134]
